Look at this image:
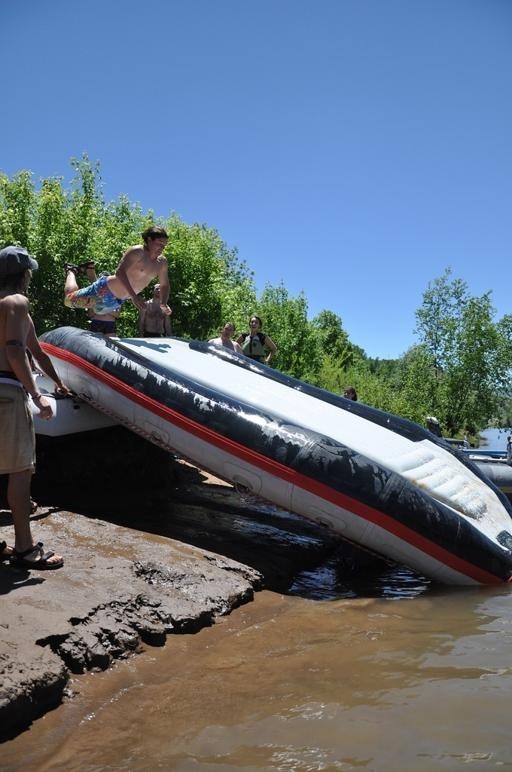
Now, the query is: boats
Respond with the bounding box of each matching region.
[24,369,119,438]
[32,323,511,588]
[457,448,511,494]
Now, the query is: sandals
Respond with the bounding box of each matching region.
[1,540,64,569]
[65,261,95,277]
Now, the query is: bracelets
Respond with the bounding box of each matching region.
[29,392,43,400]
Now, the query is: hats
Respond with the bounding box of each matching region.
[0,246,38,272]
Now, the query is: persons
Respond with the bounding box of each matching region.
[208,321,244,358]
[1,310,75,514]
[504,436,512,466]
[236,316,278,367]
[138,284,174,338]
[342,386,358,400]
[62,224,174,316]
[0,243,66,570]
[85,270,122,341]
